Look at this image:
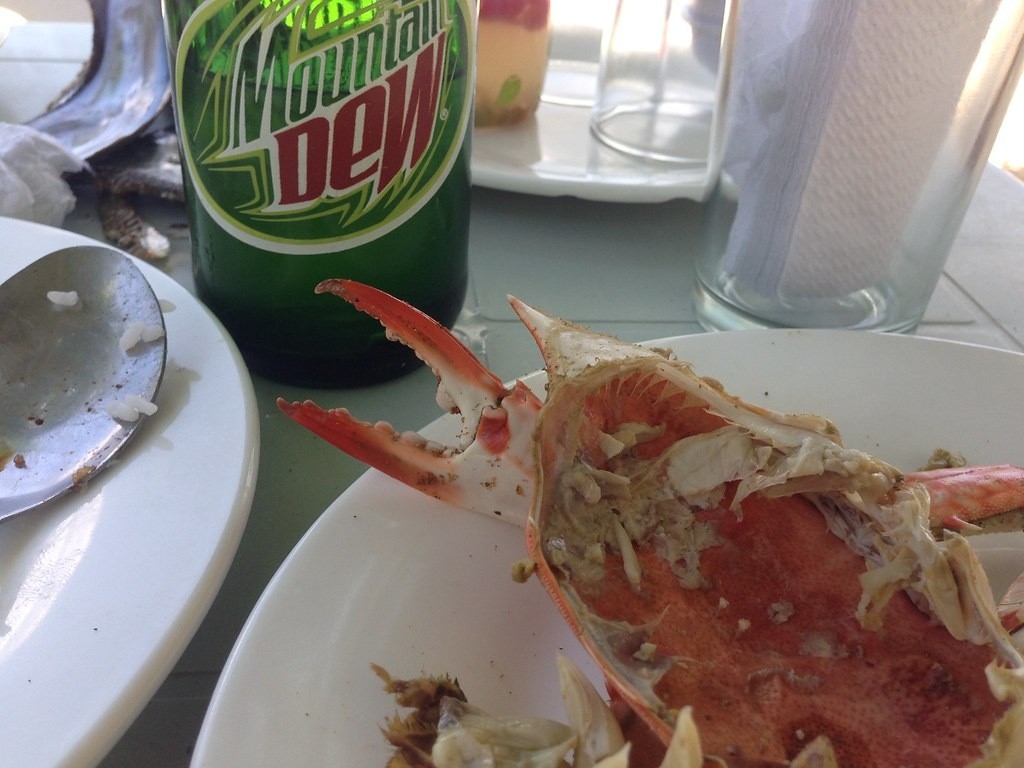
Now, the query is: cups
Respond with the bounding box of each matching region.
[472,0,724,164]
[690,1,1023,333]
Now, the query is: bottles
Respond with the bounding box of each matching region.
[162,0,469,389]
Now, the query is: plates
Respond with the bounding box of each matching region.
[0,211,263,768]
[471,60,717,204]
[184,324,1024,768]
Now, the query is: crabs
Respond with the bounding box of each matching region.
[276,275,1024,768]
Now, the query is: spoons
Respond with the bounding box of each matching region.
[0,244,169,518]
